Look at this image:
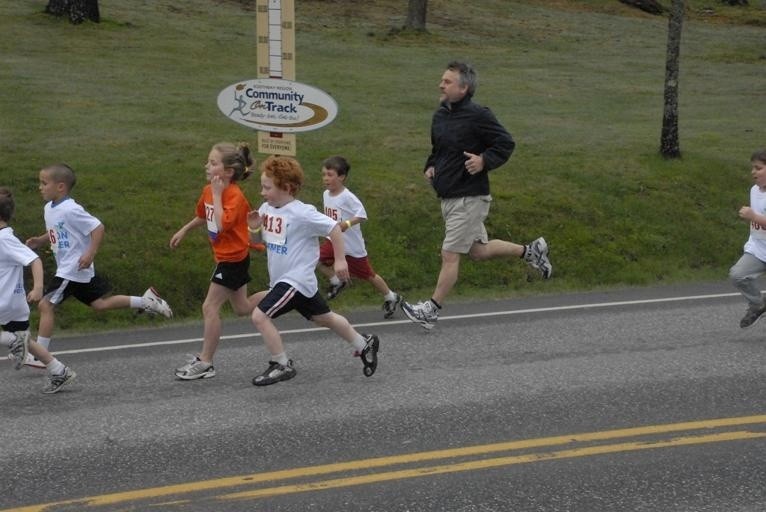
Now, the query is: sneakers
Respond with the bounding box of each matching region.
[383,292,403,319]
[326,280,346,301]
[354,332,380,377]
[174,356,216,380]
[9,353,47,369]
[251,359,298,386]
[739,296,765,329]
[142,287,173,321]
[528,236,552,280]
[40,365,78,395]
[7,329,31,371]
[399,298,440,330]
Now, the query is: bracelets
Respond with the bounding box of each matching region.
[345,220,351,228]
[248,226,262,233]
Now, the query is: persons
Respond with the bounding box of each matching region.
[170,141,270,380]
[729,149,766,330]
[315,156,403,320]
[0,189,77,394]
[9,164,173,369]
[402,60,552,331]
[246,155,380,387]
